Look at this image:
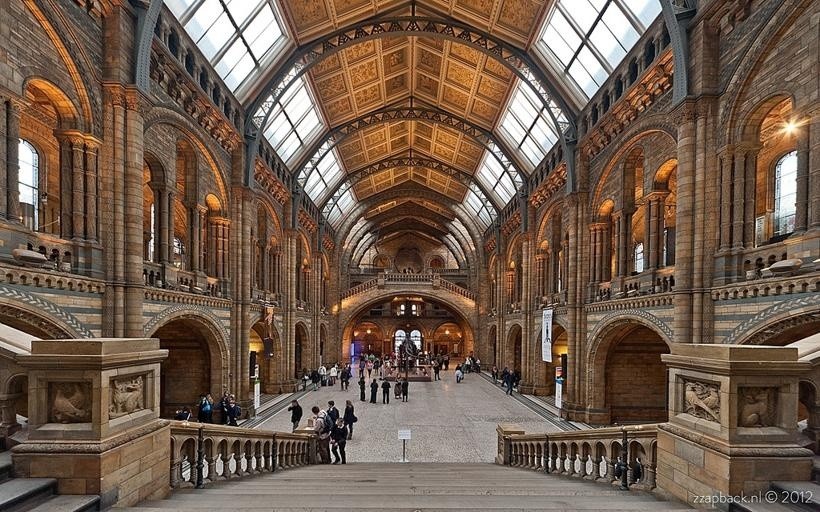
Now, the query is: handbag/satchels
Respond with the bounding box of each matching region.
[461,375,464,380]
[203,405,210,411]
[353,415,357,422]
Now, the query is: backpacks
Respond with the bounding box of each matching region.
[317,412,334,434]
[237,406,241,416]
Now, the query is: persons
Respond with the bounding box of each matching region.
[171,350,522,464]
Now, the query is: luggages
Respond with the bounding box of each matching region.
[328,377,333,385]
[322,376,326,386]
